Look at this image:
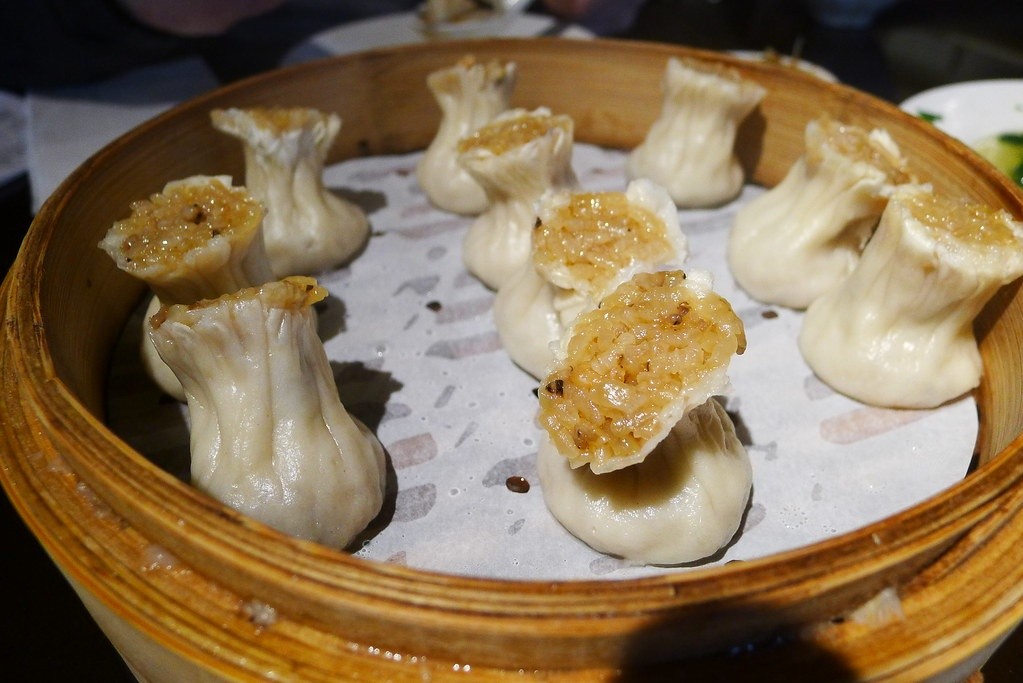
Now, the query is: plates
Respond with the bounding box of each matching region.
[897,78,1023,190]
[280,12,595,66]
